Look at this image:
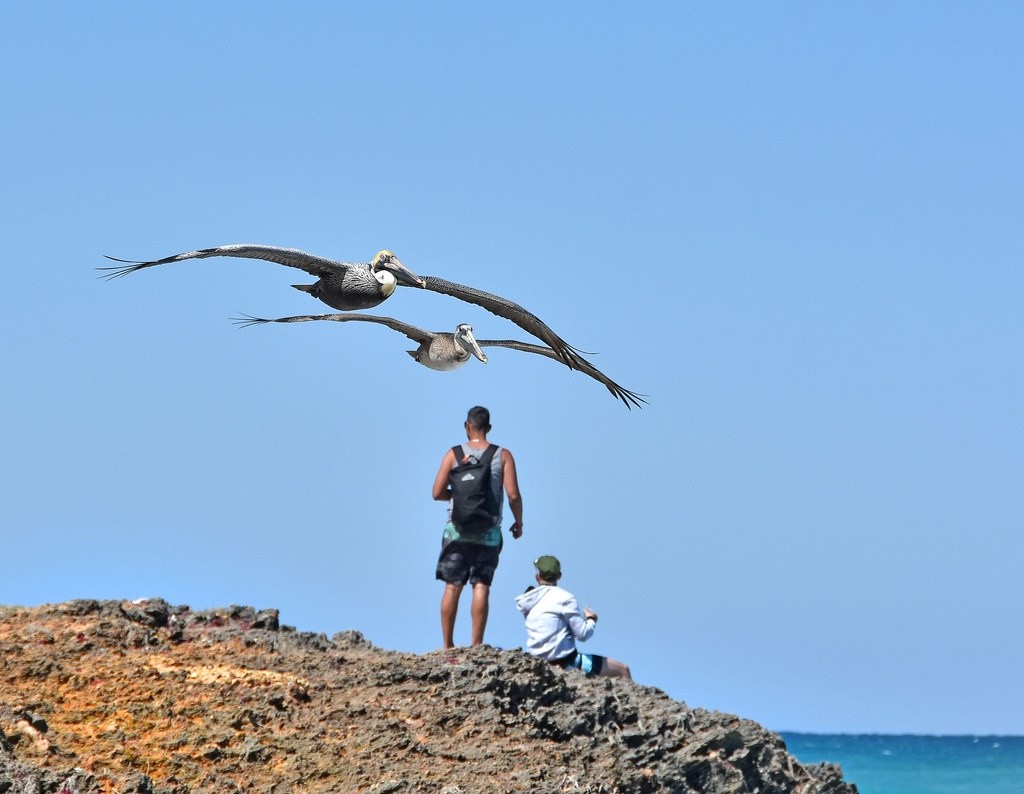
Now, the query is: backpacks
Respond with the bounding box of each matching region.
[450,444,500,534]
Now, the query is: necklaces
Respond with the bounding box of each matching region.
[469,439,486,444]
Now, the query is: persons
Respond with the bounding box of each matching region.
[432,406,523,651]
[514,554,631,681]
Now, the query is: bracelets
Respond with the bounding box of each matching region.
[587,616,596,622]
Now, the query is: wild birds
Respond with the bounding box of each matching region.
[93,242,600,372]
[227,311,653,412]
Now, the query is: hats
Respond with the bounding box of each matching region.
[534,556,560,580]
